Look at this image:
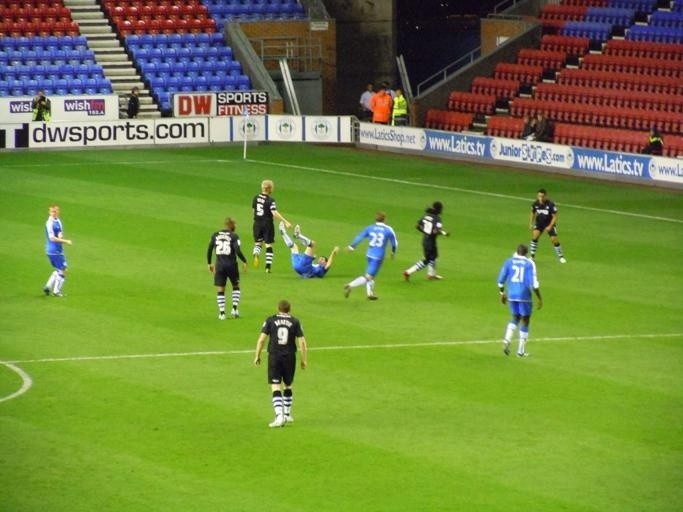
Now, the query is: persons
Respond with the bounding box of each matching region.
[42,204,74,298]
[255,300,306,427]
[402,200,451,283]
[521,116,537,141]
[342,211,399,301]
[206,216,248,321]
[251,179,294,275]
[527,188,566,263]
[535,113,551,142]
[127,87,141,120]
[278,220,340,281]
[495,244,544,361]
[641,127,663,156]
[359,81,409,126]
[31,91,53,121]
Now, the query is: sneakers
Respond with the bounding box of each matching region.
[219,314,225,320]
[266,269,271,273]
[427,274,443,279]
[503,342,511,356]
[294,225,300,238]
[559,257,566,263]
[368,295,378,300]
[344,285,351,297]
[517,353,528,358]
[42,287,64,297]
[403,271,409,281]
[231,309,239,317]
[270,415,296,426]
[279,221,286,234]
